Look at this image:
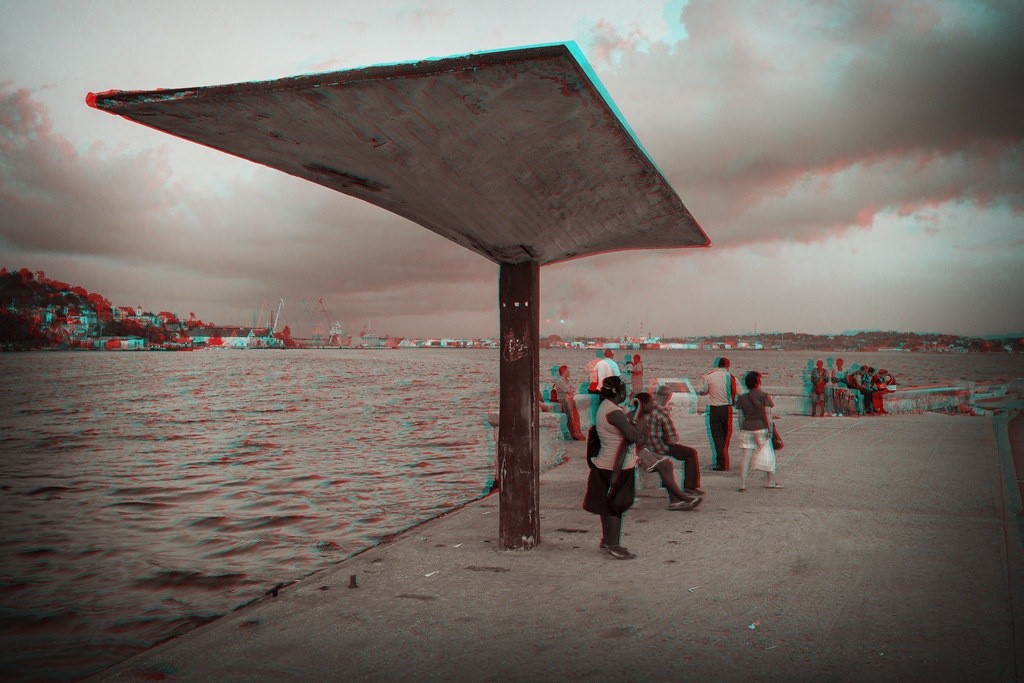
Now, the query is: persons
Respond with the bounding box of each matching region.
[700,358,744,471]
[736,371,784,492]
[648,386,706,495]
[557,365,586,441]
[594,350,621,395]
[626,355,644,402]
[810,360,829,415]
[582,375,639,558]
[831,359,849,417]
[854,365,896,416]
[627,393,702,511]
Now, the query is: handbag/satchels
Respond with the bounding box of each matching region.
[587,425,601,468]
[749,437,776,472]
[550,383,557,402]
[772,422,784,450]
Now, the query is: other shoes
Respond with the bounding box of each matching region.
[607,545,634,560]
[712,465,726,471]
[878,411,890,416]
[669,501,693,509]
[866,411,876,415]
[738,486,745,491]
[600,539,627,554]
[765,482,785,489]
[838,412,842,416]
[812,413,816,416]
[689,497,701,508]
[573,435,586,441]
[820,413,823,416]
[832,412,837,416]
[684,488,706,496]
